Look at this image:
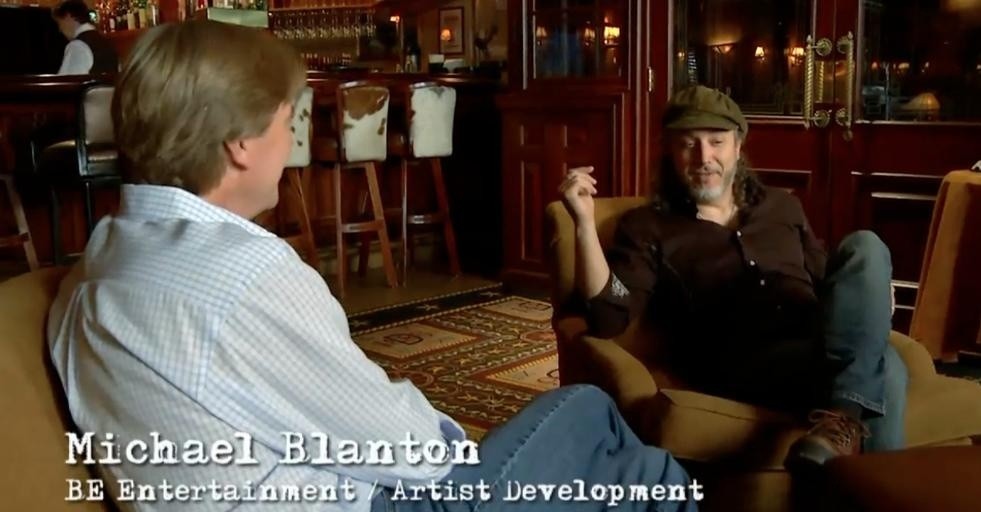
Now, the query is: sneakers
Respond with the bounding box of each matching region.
[785,411,870,483]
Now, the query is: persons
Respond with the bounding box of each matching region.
[41,15,695,512]
[48,2,121,77]
[552,81,917,481]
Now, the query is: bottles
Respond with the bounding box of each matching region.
[94,1,160,33]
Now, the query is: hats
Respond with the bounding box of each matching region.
[662,85,748,142]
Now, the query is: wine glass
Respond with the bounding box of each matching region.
[272,8,377,41]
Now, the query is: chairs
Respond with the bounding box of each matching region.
[543,189,980,512]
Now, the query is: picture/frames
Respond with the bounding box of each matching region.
[437,6,465,56]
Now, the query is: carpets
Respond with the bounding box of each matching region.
[344,282,561,447]
